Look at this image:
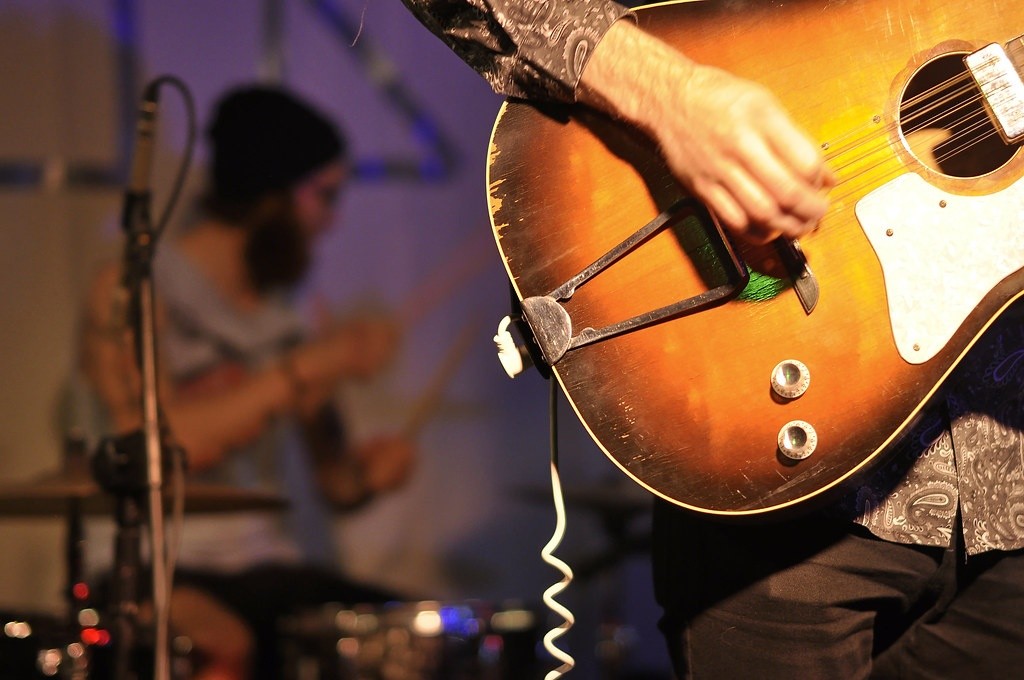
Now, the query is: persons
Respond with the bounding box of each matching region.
[398,1,1024,679]
[79,78,420,660]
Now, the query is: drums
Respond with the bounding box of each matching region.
[0,605,214,680]
[269,594,551,680]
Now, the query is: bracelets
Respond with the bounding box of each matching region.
[282,352,307,395]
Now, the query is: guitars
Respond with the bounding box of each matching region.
[484,0,1024,517]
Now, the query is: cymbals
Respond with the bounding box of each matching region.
[0,470,297,523]
[498,477,652,520]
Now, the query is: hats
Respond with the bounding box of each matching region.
[212,86,341,206]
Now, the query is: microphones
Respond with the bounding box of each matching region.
[119,84,159,228]
